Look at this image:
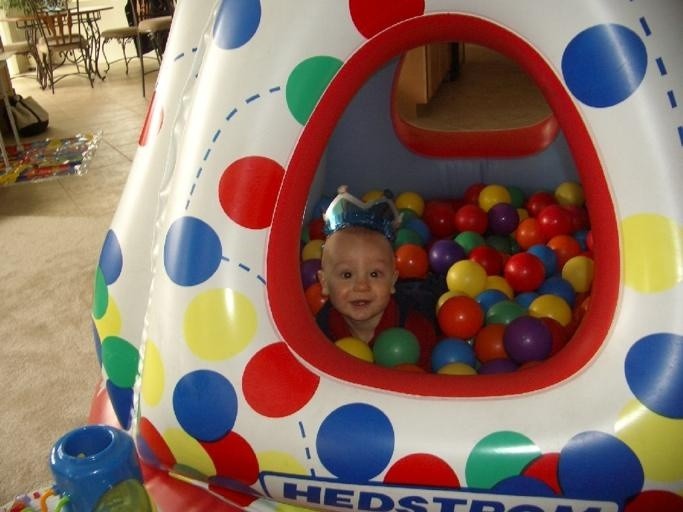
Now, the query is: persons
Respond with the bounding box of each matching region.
[315,192,448,358]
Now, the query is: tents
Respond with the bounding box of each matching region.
[88,0,683,512]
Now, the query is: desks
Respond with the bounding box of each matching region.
[0,6,116,73]
[0,51,23,175]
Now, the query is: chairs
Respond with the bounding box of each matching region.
[100,0,136,74]
[29,0,96,93]
[132,1,172,98]
[0,35,47,89]
